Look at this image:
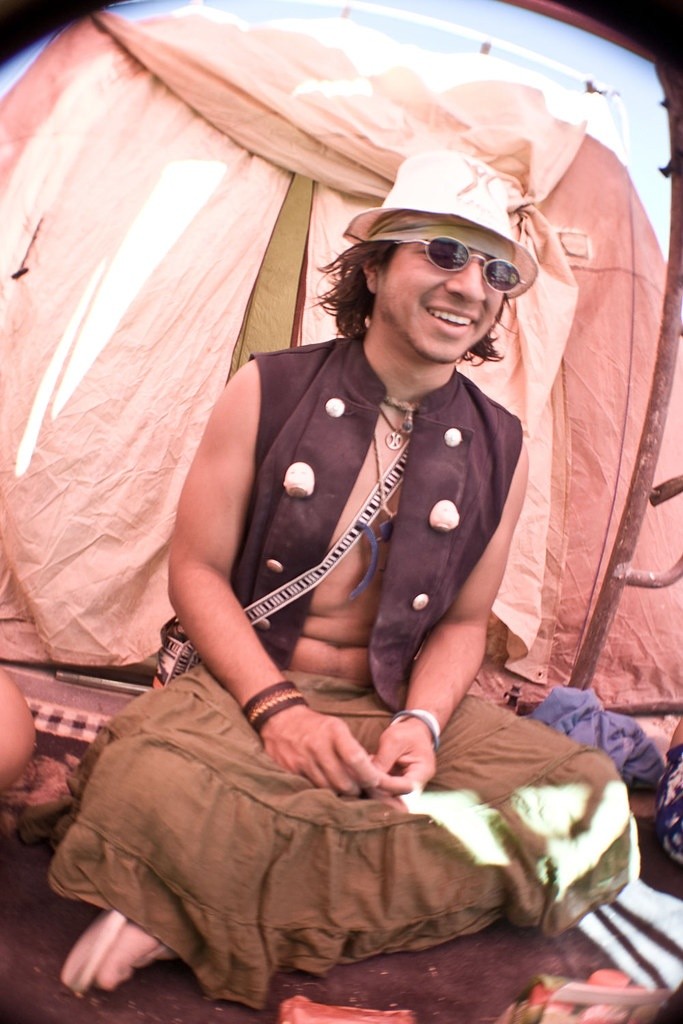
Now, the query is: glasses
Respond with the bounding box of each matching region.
[393,236,527,293]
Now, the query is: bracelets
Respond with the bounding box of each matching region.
[242,680,310,733]
[387,709,441,753]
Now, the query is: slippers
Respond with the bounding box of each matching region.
[58,897,166,994]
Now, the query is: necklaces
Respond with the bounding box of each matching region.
[344,394,422,602]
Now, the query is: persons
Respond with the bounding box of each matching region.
[653,716,683,864]
[46,149,633,990]
[0,667,37,788]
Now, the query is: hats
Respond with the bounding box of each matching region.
[343,149,539,300]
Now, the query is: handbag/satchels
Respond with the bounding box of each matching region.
[152,612,202,691]
